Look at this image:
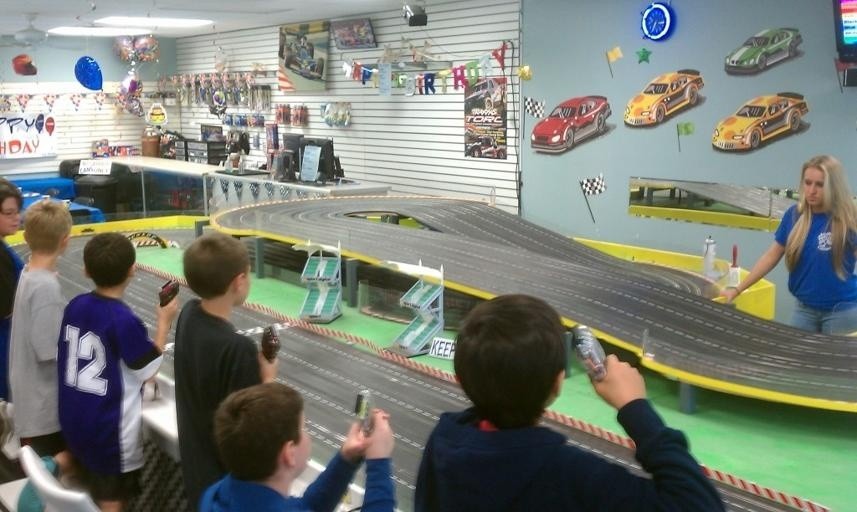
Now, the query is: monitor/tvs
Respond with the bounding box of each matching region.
[833,0,857,63]
[281,133,305,181]
[300,138,334,186]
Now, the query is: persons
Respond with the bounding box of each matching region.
[173,228,280,511]
[415,293,727,511]
[201,380,397,512]
[720,153,857,336]
[7,197,75,480]
[17,231,178,511]
[0,177,25,450]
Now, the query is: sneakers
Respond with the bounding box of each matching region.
[15,455,62,512]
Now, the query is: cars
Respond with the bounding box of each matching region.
[529,94,614,154]
[711,91,810,151]
[724,26,804,75]
[624,69,707,127]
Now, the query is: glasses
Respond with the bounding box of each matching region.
[2,206,26,218]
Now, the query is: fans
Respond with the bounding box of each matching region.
[0,28,85,51]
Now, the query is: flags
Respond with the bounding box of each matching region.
[608,46,623,63]
[835,58,856,72]
[678,121,695,136]
[581,174,607,195]
[523,96,546,118]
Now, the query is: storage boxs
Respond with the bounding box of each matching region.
[174,138,226,164]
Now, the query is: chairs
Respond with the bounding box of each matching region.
[0,443,100,512]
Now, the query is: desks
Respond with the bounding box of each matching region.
[205,170,392,214]
[8,178,106,224]
[102,159,207,219]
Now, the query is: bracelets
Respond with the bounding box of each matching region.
[735,287,740,294]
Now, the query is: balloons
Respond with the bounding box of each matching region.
[11,54,38,77]
[74,55,104,94]
[112,35,161,118]
